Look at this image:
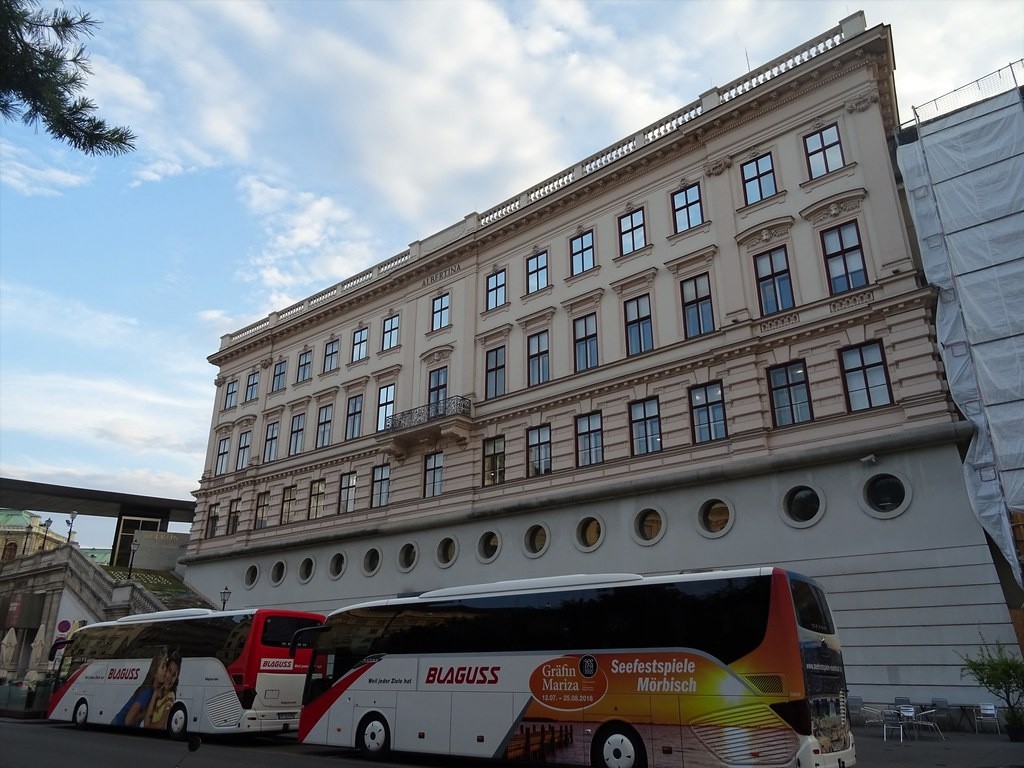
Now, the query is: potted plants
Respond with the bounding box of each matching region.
[953,631,1024,742]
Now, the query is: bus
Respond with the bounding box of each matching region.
[47,608,333,741]
[289,566,857,768]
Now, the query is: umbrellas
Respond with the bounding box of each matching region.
[24,625,46,680]
[0,628,17,679]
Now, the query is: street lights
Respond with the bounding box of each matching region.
[128,539,140,579]
[220,586,232,610]
[22,524,33,554]
[2,535,10,560]
[43,517,53,549]
[66,510,78,542]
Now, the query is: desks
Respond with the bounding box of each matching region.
[881,708,916,740]
[948,704,980,733]
[909,703,936,731]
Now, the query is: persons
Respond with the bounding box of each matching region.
[144,652,182,730]
[111,647,167,727]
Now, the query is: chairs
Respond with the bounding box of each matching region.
[881,710,905,743]
[848,696,867,726]
[900,705,920,741]
[861,706,888,740]
[973,703,1000,735]
[927,698,954,734]
[915,709,945,744]
[887,697,910,731]
